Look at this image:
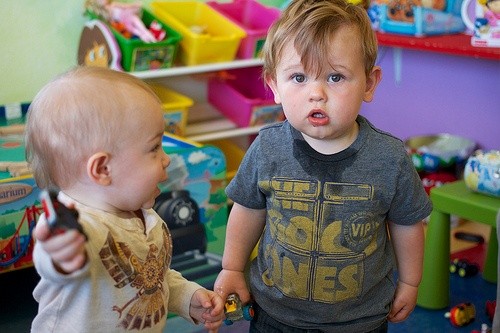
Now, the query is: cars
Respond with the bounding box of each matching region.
[444,301,477,328]
[39,188,91,240]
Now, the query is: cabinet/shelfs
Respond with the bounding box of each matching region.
[82,24,283,205]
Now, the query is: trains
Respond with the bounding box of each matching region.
[152,189,207,255]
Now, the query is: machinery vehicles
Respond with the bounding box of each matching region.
[449,258,480,278]
[222,292,255,325]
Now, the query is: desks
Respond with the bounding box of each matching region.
[417,180,500,309]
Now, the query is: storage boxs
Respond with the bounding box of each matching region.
[149,0,247,68]
[207,66,284,127]
[85,0,183,71]
[146,83,194,139]
[201,141,246,183]
[206,0,282,59]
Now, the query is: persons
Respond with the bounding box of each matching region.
[22,65,224,332]
[214,0,433,333]
